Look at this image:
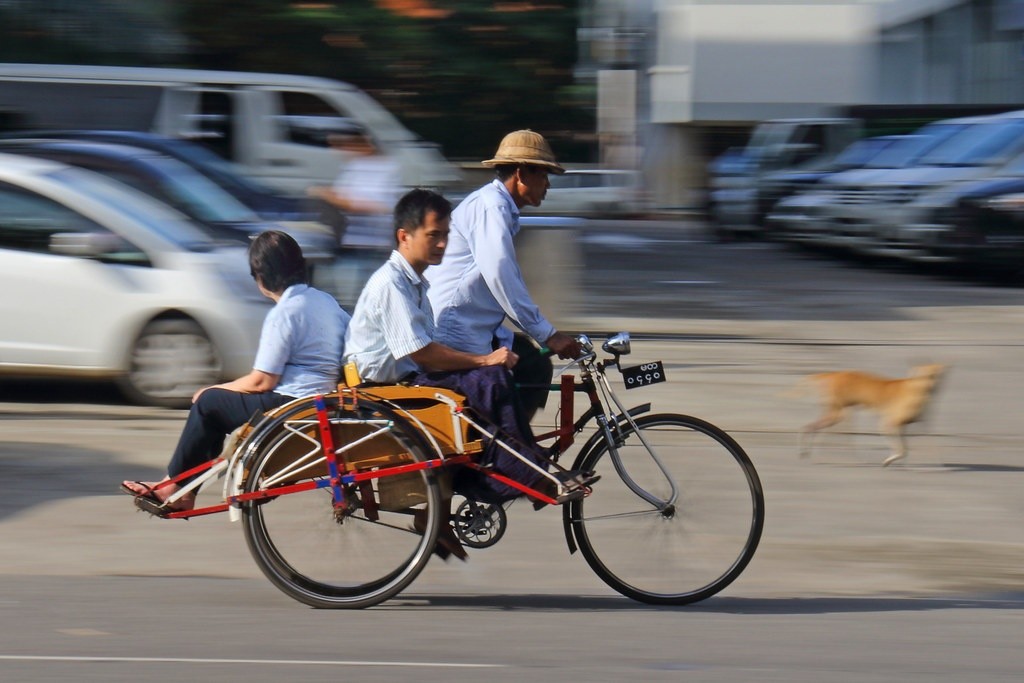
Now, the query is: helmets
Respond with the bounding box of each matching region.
[481,129,566,176]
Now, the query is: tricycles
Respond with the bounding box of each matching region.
[133,329,765,613]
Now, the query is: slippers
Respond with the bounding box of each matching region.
[118,481,189,521]
[412,521,467,561]
[531,470,602,511]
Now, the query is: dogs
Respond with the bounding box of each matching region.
[787,362,951,466]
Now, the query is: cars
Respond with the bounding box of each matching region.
[709,110,1024,285]
[0,62,483,408]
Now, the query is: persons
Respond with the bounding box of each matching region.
[118,231,354,523]
[414,129,582,561]
[308,120,400,320]
[338,189,601,509]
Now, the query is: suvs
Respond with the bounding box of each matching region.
[523,166,660,219]
